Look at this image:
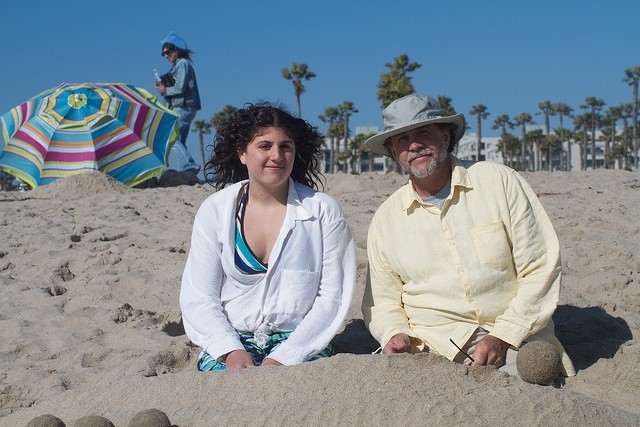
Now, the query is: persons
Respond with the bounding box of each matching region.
[360,93,578,380]
[179,101,357,372]
[153,31,202,177]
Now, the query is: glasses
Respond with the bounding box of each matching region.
[161,50,170,56]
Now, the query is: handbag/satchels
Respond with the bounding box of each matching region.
[160,73,176,109]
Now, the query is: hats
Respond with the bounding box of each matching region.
[363,93,467,158]
[161,31,187,50]
